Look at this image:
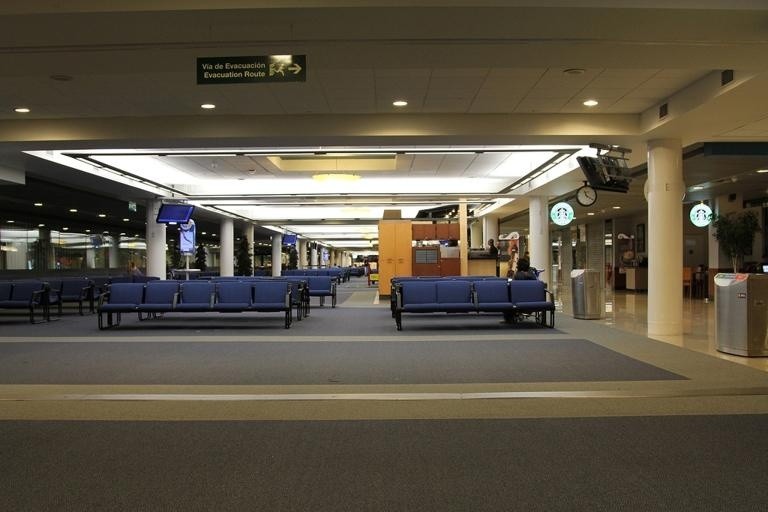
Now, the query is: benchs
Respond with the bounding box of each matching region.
[389,275,555,331]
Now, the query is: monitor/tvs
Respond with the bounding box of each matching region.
[156,204,196,224]
[283,235,297,246]
[577,142,632,194]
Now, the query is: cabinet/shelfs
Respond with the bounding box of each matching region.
[435,223,460,241]
[412,223,436,240]
[412,244,441,276]
[378,219,412,296]
[625,268,647,290]
[440,257,460,276]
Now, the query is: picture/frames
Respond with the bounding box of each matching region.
[636,223,645,252]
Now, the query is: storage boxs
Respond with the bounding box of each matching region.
[383,210,401,219]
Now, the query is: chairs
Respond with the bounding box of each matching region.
[683,267,692,298]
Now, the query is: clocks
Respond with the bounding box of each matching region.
[575,185,597,207]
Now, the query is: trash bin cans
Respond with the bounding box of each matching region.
[571,269,602,320]
[713,273,768,356]
